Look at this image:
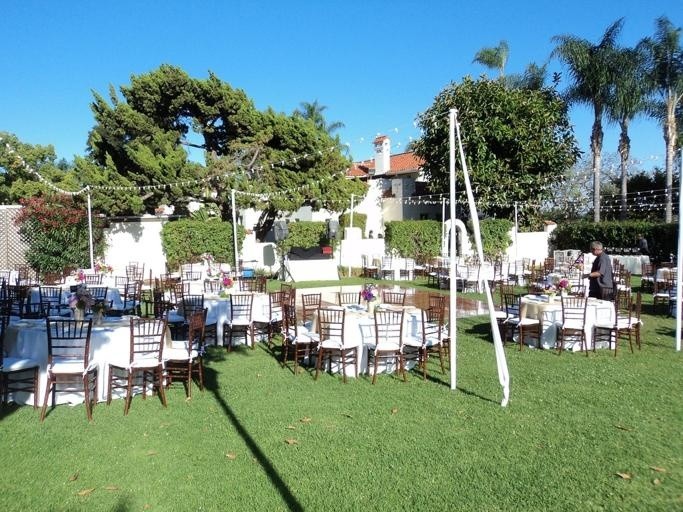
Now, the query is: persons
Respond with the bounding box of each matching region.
[636,234,650,255]
[580,240,615,301]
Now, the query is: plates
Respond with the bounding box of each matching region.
[121,315,140,320]
[12,316,62,328]
